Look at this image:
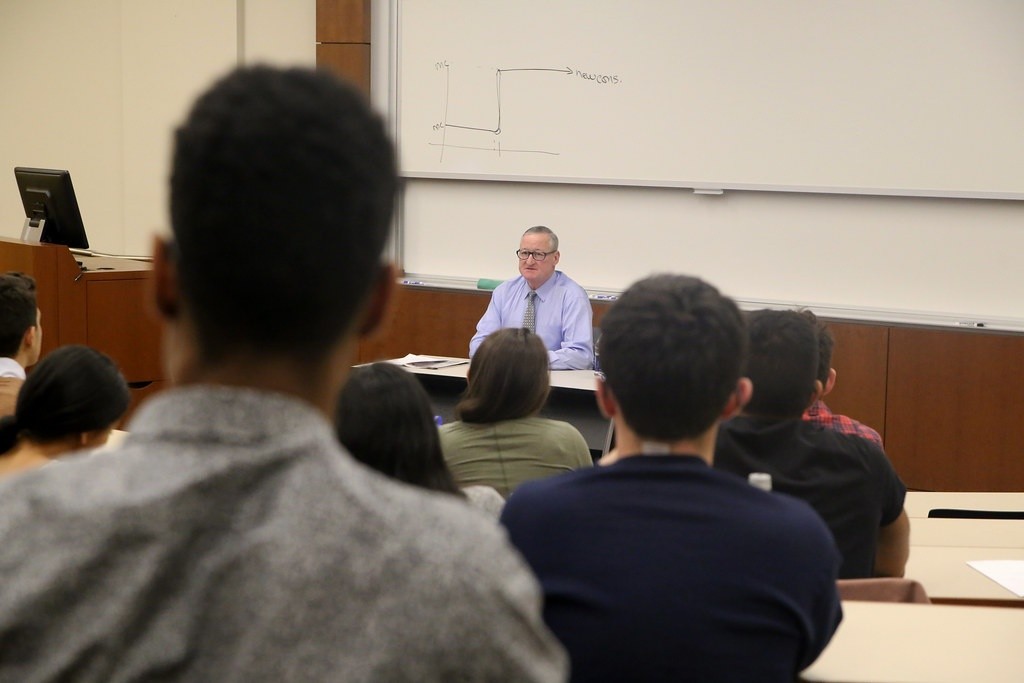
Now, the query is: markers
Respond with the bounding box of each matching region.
[950,322,985,327]
[402,280,423,285]
[591,294,617,299]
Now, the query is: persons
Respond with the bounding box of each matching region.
[437,329,595,500]
[499,274,841,683]
[469,227,594,369]
[0,64,569,681]
[331,363,504,521]
[2,346,129,489]
[711,308,908,579]
[800,312,885,448]
[1,272,43,415]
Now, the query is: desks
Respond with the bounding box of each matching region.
[0,237,172,381]
[790,491,1024,682]
[352,353,617,469]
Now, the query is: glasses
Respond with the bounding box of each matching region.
[516,249,557,261]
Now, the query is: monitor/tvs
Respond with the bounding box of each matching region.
[14,167,89,250]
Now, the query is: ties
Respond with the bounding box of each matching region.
[521,292,538,335]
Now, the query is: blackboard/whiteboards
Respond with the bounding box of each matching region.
[390,0,1024,200]
